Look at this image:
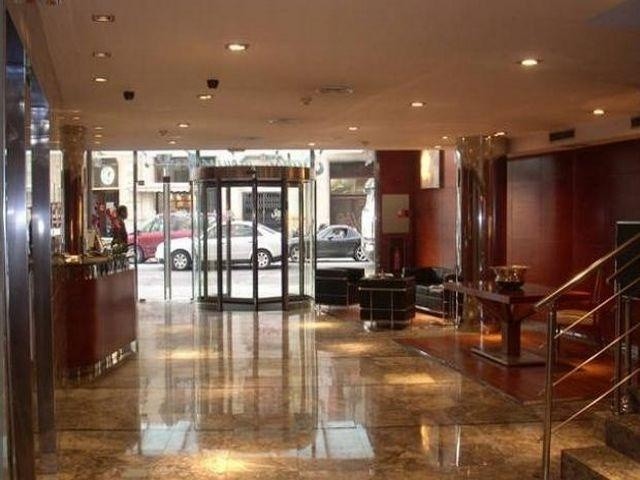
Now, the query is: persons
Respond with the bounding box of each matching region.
[113,205,128,252]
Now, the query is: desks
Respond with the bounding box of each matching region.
[444,278,592,367]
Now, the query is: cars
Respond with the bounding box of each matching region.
[125,211,368,271]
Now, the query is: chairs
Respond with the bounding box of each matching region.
[316,268,416,333]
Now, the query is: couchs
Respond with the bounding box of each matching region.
[402,265,457,316]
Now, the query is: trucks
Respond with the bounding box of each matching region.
[361,176,376,263]
